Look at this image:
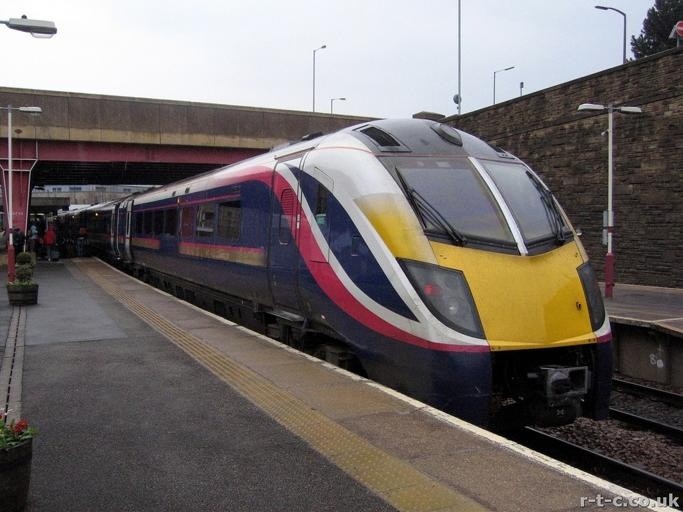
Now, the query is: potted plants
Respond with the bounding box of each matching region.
[7,252,37,306]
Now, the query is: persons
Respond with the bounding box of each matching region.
[7,227,25,255]
[27,225,38,251]
[42,225,56,262]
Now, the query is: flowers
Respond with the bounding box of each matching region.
[0,408,37,449]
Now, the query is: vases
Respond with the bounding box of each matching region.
[0,439,32,511]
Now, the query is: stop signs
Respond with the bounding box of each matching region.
[676,21,683,37]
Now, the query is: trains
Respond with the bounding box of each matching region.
[46,117,616,435]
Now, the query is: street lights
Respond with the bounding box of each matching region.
[312,45,327,112]
[492,66,515,106]
[595,5,626,65]
[331,98,346,115]
[0,14,58,283]
[577,103,641,298]
